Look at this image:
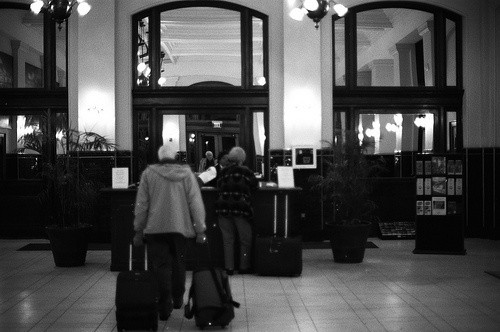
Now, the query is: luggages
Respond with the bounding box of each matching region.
[184,243,234,329]
[257,190,302,277]
[115,238,158,332]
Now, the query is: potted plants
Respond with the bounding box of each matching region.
[13,108,120,268]
[307,132,378,263]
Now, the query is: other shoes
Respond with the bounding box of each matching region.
[227,268,235,274]
[174,301,183,309]
[160,313,170,320]
[239,268,255,274]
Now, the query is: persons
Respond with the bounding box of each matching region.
[213,147,260,276]
[132,144,206,321]
[199,151,218,172]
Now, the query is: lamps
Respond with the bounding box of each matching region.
[30,0,91,32]
[288,0,348,29]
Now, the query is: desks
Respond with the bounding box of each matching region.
[99,181,139,272]
[201,182,303,266]
